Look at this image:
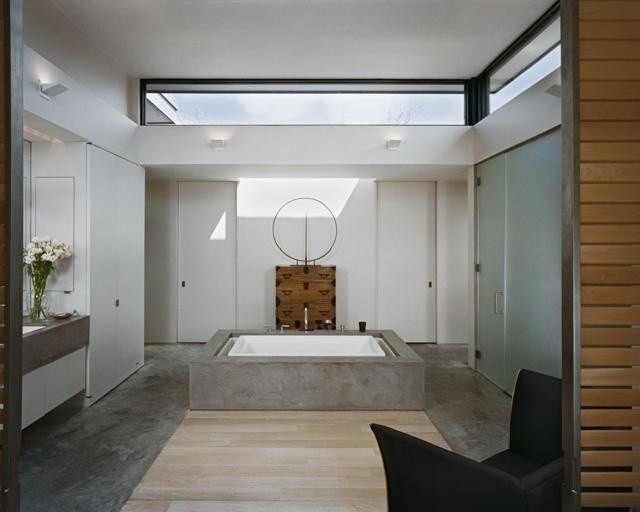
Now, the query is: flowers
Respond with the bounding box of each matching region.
[21,233,73,319]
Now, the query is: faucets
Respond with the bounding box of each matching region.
[303,306,309,331]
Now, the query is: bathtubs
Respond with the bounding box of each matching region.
[210,332,402,363]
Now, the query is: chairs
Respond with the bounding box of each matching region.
[370,368,631,512]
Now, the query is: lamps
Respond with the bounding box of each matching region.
[38,78,66,101]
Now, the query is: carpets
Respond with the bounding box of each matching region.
[121,409,453,511]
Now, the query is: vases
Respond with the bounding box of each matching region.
[29,288,48,321]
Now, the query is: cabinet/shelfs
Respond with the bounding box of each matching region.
[275,265,336,330]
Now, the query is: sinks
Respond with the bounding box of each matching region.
[22,322,45,335]
